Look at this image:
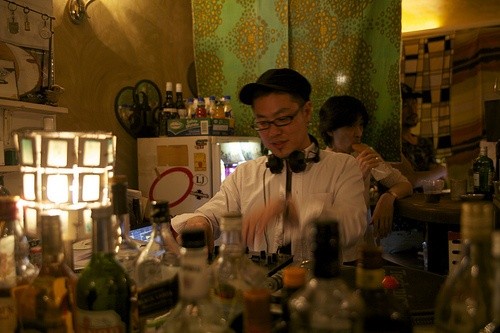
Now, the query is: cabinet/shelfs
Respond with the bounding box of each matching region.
[0,99,69,171]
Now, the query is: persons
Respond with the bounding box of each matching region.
[380,82,436,265]
[172,68,367,262]
[318,95,412,242]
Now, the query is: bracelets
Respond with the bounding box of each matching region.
[386,191,397,199]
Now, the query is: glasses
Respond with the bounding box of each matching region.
[252,105,304,131]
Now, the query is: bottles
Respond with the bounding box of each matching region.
[162,81,234,120]
[473,145,494,198]
[0,175,499,333]
[353,139,393,180]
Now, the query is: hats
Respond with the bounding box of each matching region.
[239,68,317,105]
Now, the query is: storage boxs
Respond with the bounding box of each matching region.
[166,118,230,135]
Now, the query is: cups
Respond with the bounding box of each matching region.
[3,148,18,165]
[449,179,467,201]
[423,180,444,203]
[42,116,54,132]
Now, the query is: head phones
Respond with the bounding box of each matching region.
[265,134,320,175]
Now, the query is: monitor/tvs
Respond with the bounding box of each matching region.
[131,223,167,258]
